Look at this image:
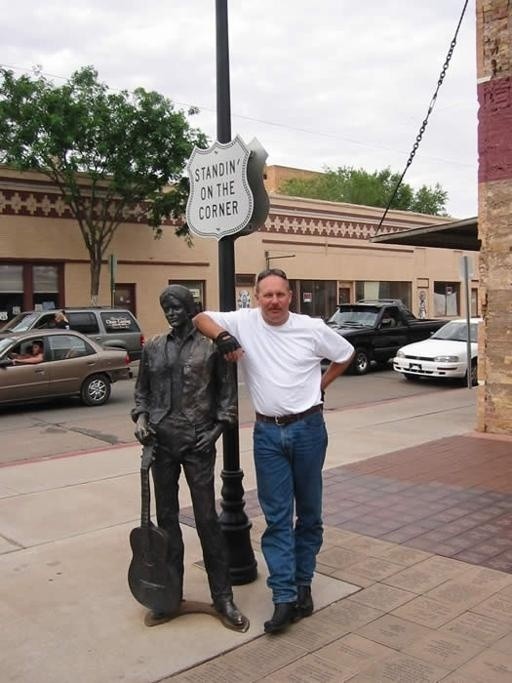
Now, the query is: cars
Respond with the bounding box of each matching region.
[392,318,478,387]
[0,329,132,407]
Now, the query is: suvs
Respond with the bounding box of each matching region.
[0,306,146,364]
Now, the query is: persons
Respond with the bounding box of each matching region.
[36,309,69,328]
[130,284,244,625]
[193,267,357,633]
[5,340,44,365]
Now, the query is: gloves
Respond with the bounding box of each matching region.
[213,331,244,357]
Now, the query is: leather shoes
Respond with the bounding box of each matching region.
[295,582,314,618]
[263,600,300,634]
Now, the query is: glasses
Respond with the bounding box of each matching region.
[256,268,287,284]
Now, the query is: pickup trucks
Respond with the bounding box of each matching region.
[320,298,451,376]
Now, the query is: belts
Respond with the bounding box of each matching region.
[254,403,323,426]
